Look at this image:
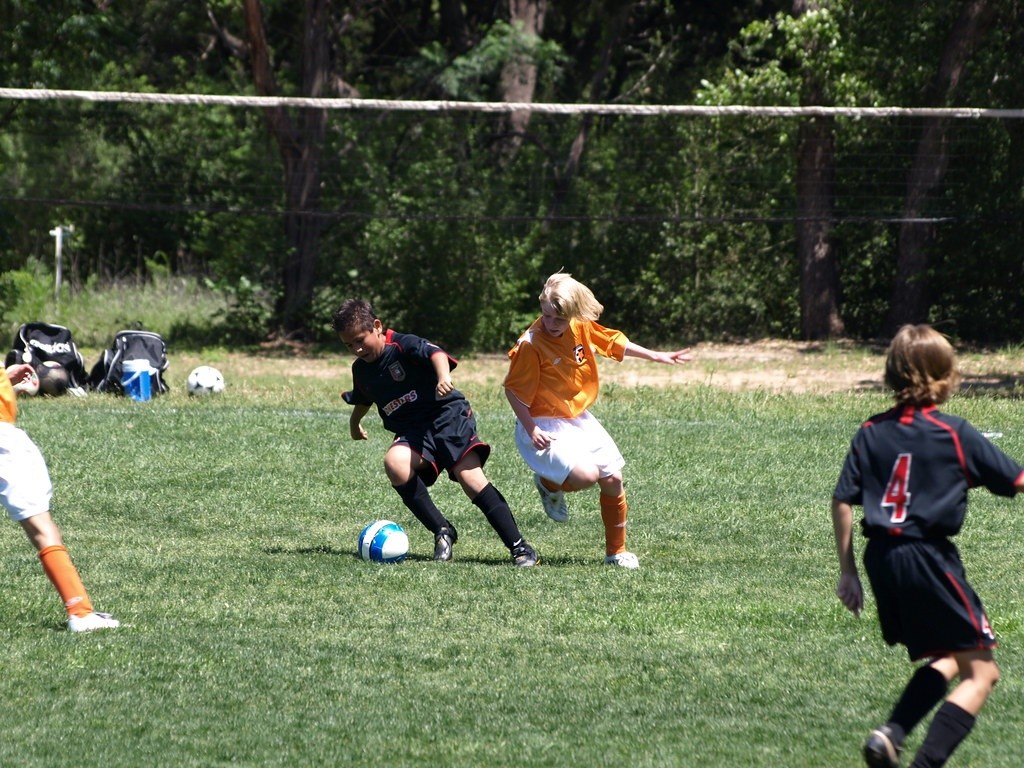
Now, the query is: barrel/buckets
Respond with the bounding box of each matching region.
[120,359,152,400]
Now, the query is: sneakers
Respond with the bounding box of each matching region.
[604,551,639,569]
[68,612,120,632]
[433,519,458,561]
[533,472,568,523]
[863,725,904,768]
[511,544,540,567]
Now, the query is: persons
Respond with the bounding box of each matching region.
[329,298,535,574]
[0,361,119,633]
[501,266,693,572]
[829,322,1024,768]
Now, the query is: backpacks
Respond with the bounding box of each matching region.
[89,321,170,398]
[4,321,93,397]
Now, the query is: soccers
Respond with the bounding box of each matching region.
[184,364,227,401]
[358,521,410,561]
[6,361,39,399]
[37,359,69,395]
